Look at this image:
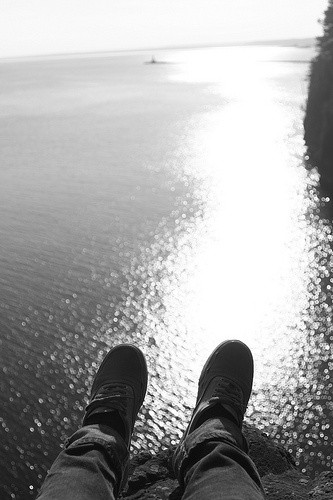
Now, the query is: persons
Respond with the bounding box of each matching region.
[33,339,267,500]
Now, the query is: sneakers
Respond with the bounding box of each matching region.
[173,339,255,466]
[82,344,148,487]
